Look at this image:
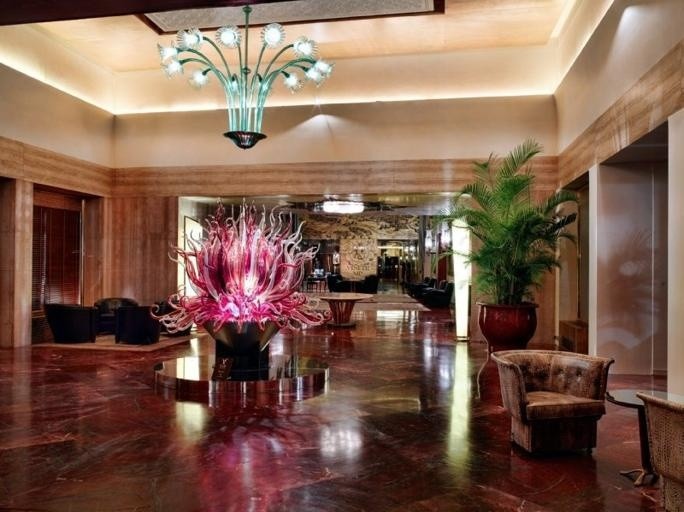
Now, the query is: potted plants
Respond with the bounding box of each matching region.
[428,135,583,347]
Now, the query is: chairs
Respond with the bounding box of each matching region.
[633,389,684,512]
[409,276,454,309]
[328,272,379,294]
[490,346,617,462]
[42,297,194,346]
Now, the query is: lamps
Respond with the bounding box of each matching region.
[156,6,336,151]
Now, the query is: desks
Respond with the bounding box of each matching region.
[602,385,683,487]
[320,291,374,327]
